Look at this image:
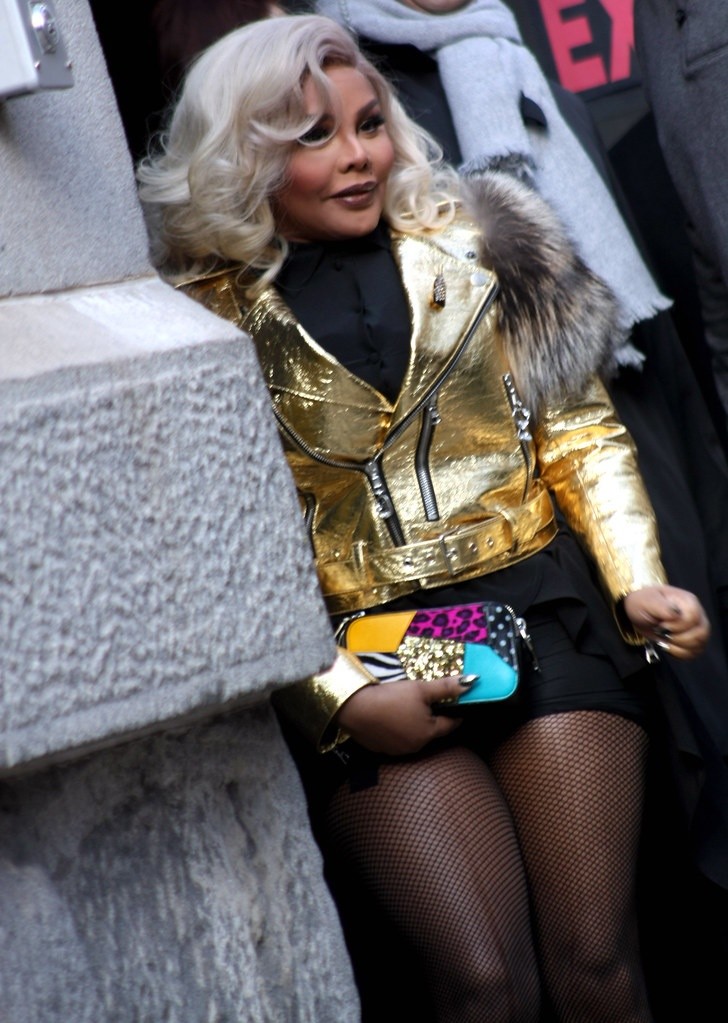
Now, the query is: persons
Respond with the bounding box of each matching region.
[135,14,710,1023]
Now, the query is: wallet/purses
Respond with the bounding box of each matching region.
[341,601,531,717]
